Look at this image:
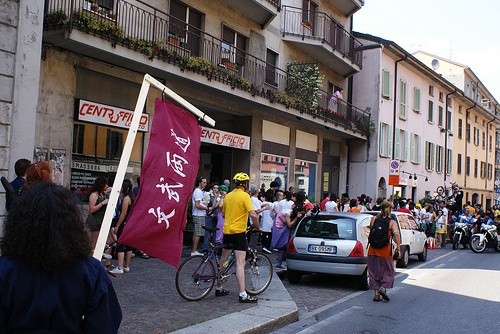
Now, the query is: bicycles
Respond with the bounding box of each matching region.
[175,225,274,301]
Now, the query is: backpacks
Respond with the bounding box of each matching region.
[368,216,392,248]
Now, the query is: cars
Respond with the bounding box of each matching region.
[282,206,375,290]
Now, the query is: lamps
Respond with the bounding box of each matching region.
[401,170,428,182]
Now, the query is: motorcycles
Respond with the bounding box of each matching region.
[469,217,500,253]
[453,217,475,250]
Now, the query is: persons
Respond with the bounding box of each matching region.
[366,200,401,302]
[87,176,149,274]
[328,88,344,113]
[26,161,52,182]
[189,177,500,271]
[0,182,122,334]
[5,159,31,211]
[214,171,259,303]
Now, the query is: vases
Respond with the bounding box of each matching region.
[85,0,114,20]
[222,59,237,68]
[169,36,181,46]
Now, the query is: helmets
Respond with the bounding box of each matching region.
[232,172,250,185]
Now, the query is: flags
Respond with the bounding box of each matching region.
[117,97,202,268]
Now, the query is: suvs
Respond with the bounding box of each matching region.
[360,211,429,268]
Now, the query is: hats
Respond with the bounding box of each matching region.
[217,185,228,193]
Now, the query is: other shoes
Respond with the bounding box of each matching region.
[131,253,136,258]
[190,251,204,257]
[262,247,272,254]
[215,288,230,297]
[377,286,390,302]
[373,294,384,301]
[141,252,150,259]
[239,294,258,303]
[104,263,115,267]
[108,266,130,274]
[275,265,287,270]
[274,248,279,251]
